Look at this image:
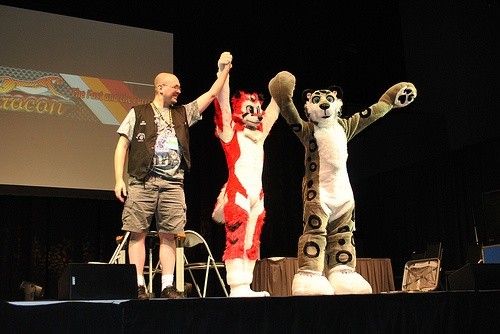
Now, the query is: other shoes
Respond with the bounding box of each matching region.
[137,285,149,300]
[160,285,182,298]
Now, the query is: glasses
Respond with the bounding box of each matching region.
[162,84,181,91]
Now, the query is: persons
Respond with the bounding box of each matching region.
[113,63,231,300]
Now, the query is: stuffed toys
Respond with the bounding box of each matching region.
[212,52,280,297]
[268,71,418,296]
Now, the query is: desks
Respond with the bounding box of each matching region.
[116,231,186,298]
[251,257,395,297]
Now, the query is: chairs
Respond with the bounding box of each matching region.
[185,230,229,298]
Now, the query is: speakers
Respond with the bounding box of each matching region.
[447,263,500,290]
[58,263,137,300]
